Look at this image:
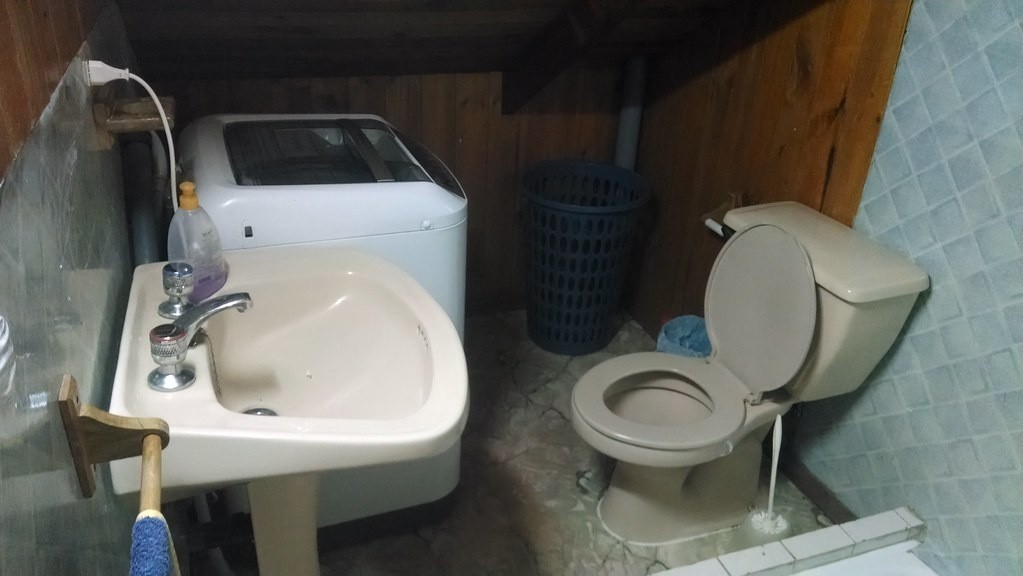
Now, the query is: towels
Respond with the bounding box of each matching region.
[125,508,185,576]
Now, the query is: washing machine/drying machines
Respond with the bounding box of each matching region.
[164,112,468,547]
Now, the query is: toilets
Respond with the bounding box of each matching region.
[566,201,931,551]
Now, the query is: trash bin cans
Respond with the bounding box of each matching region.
[658,313,712,358]
[517,159,657,357]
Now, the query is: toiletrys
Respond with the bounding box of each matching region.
[166,180,227,307]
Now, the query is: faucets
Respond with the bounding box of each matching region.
[166,292,256,355]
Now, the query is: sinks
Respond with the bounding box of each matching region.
[101,246,472,507]
